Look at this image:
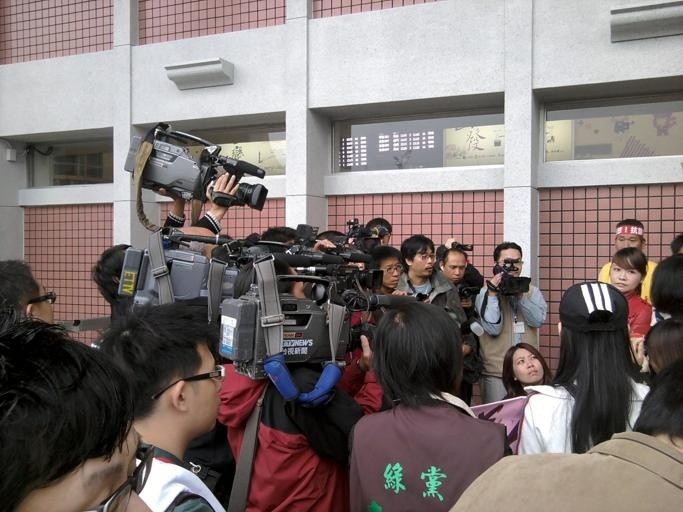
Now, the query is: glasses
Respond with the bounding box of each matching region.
[379,262,403,275]
[413,253,436,262]
[150,363,226,405]
[25,290,58,307]
[88,444,157,511]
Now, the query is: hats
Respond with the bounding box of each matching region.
[558,280,630,334]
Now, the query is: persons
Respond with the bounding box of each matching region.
[671,232,683,254]
[364,217,392,246]
[329,311,384,417]
[0,313,155,512]
[556,246,653,372]
[0,258,57,326]
[474,241,548,404]
[314,230,348,251]
[438,248,485,407]
[512,280,650,455]
[629,316,683,389]
[435,245,484,289]
[446,357,682,512]
[397,235,467,329]
[91,301,229,511]
[261,226,298,245]
[360,243,416,328]
[348,300,513,512]
[597,218,657,307]
[214,252,384,512]
[93,171,241,331]
[649,252,683,327]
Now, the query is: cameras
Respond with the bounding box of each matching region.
[450,238,475,254]
[343,218,374,239]
[496,256,532,295]
[408,290,428,302]
[452,281,482,300]
